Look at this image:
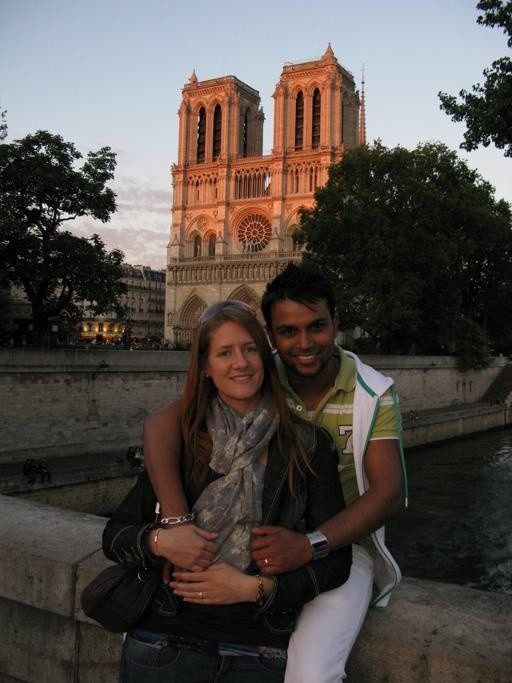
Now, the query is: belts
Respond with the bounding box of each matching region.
[131,628,288,661]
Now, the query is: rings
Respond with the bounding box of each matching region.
[199,590,204,601]
[264,558,272,569]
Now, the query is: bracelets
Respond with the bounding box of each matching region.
[159,513,195,526]
[306,530,331,564]
[153,527,162,558]
[256,572,264,607]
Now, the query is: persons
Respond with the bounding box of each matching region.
[102,300,353,681]
[140,262,412,681]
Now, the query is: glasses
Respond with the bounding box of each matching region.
[198,300,257,324]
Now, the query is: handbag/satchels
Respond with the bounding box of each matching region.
[81,564,159,634]
